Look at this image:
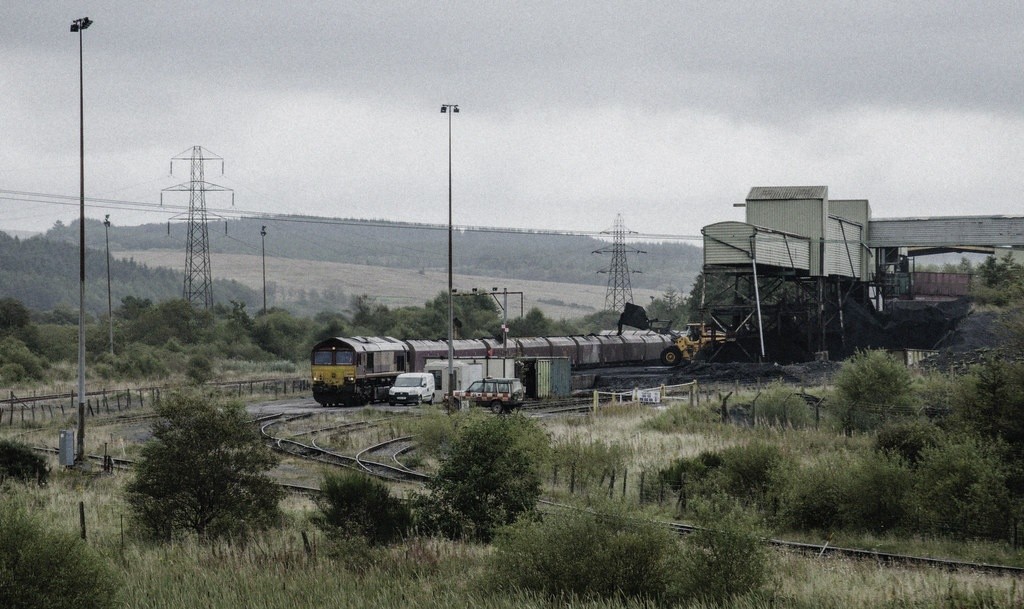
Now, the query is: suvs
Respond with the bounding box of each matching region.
[443,377,527,414]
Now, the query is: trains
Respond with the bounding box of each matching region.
[311,329,687,406]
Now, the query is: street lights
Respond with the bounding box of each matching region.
[440,103,458,410]
[103,215,114,355]
[260,226,266,312]
[70,17,94,458]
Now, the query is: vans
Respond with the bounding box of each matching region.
[388,373,436,406]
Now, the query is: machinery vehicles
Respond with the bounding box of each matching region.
[620,302,735,366]
